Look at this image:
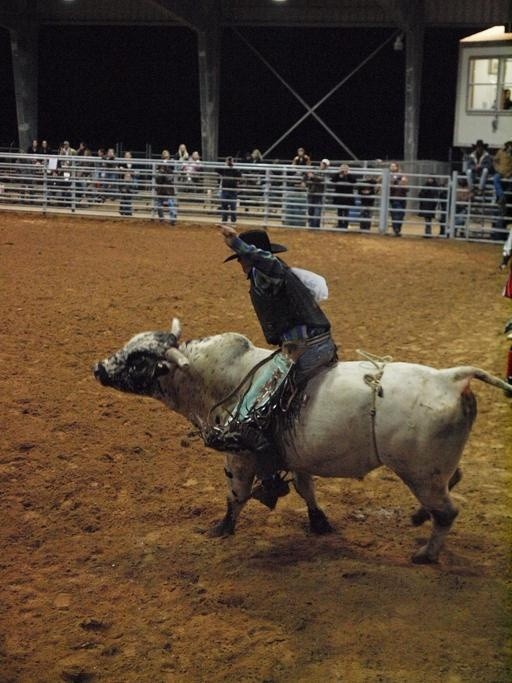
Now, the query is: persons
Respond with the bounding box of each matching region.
[504,89,511,108]
[205,222,340,507]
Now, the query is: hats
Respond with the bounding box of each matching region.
[471,139,489,149]
[220,229,288,264]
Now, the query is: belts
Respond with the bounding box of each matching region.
[304,329,331,346]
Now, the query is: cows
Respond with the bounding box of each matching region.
[92,317,512,565]
